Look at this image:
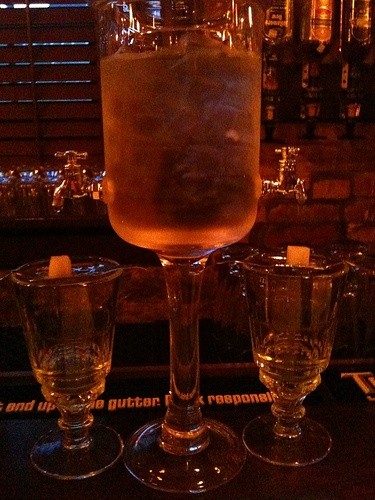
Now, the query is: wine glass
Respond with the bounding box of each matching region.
[242,253,350,467]
[10,256,123,480]
[97,0,265,493]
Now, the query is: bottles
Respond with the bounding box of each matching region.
[264,0,375,146]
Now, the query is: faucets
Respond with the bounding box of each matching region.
[262,146,309,207]
[52,150,101,207]
